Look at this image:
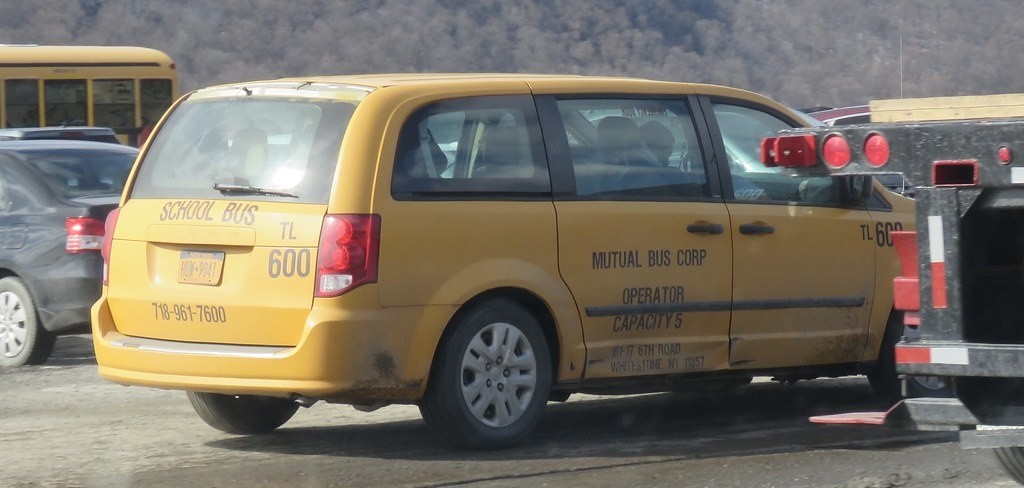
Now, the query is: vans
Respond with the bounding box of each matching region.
[92,72,915,449]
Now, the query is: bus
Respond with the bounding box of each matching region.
[0,40,177,148]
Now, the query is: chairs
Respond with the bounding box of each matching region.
[474,126,529,180]
[597,115,661,166]
[230,128,277,190]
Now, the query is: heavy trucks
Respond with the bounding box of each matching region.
[760,90,1024,487]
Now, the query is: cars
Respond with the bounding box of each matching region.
[0,126,142,370]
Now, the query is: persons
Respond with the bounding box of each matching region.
[640,121,675,168]
